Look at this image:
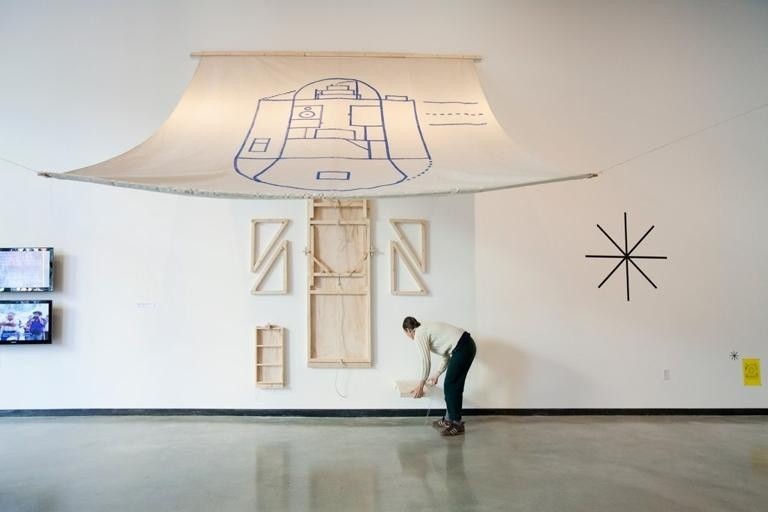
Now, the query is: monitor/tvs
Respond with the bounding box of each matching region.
[0,300,52,344]
[0,247,54,292]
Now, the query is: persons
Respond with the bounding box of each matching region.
[403,315,478,436]
[0,310,51,340]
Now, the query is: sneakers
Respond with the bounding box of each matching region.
[433,417,465,436]
